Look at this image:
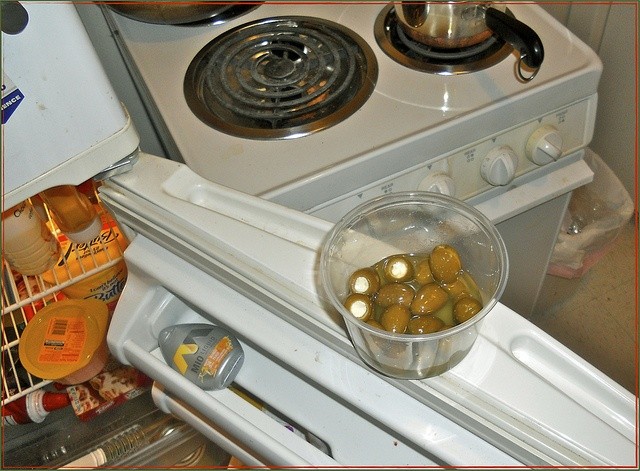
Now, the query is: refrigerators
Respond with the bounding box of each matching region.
[0,0,640,470]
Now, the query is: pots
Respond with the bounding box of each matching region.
[102,0,237,25]
[393,0,544,83]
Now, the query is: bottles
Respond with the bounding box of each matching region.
[1,197,62,277]
[37,183,102,242]
[1,387,70,429]
[157,322,246,392]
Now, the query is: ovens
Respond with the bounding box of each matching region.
[384,149,595,321]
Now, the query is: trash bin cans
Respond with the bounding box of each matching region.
[546,147,635,280]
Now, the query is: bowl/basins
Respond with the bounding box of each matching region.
[320,188,511,380]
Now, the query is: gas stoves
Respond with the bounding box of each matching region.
[92,0,603,212]
